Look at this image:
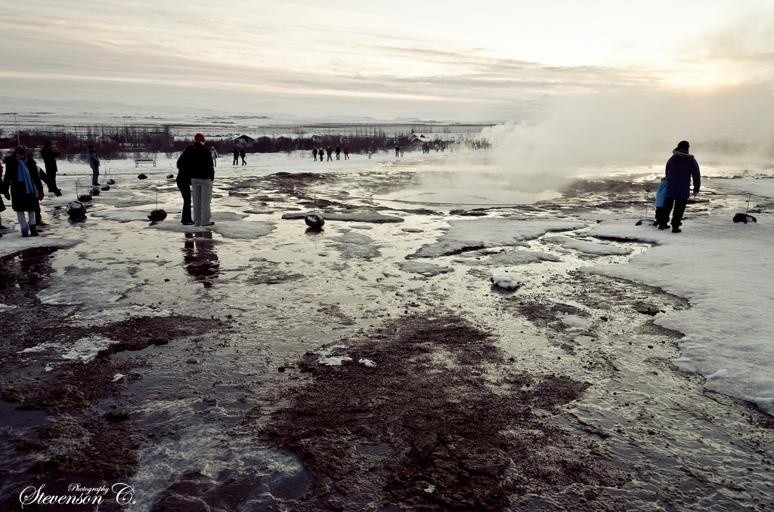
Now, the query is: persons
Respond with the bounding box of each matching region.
[0,140,62,237]
[231,149,239,165]
[240,148,246,166]
[176,146,194,225]
[88,144,100,185]
[335,145,341,160]
[190,133,215,226]
[395,144,400,157]
[343,143,349,160]
[209,146,217,167]
[660,140,700,232]
[312,146,317,161]
[654,178,667,227]
[326,146,333,162]
[318,148,324,161]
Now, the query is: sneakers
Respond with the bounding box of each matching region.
[657,221,683,234]
[21,221,49,239]
